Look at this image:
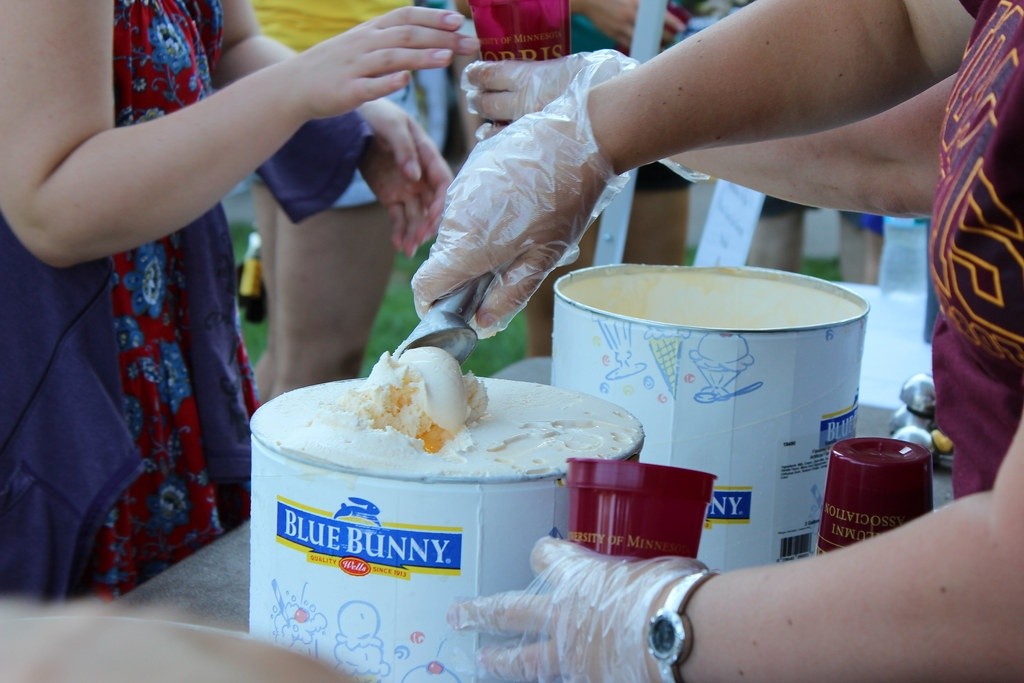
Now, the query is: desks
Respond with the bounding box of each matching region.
[104,358,957,636]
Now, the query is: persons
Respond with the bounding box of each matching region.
[0,0,478,611]
[461,48,958,217]
[255,0,415,399]
[446,0,690,358]
[411,0,1023,683]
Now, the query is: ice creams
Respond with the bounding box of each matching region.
[251,346,644,477]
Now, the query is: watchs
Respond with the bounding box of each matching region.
[647,571,716,683]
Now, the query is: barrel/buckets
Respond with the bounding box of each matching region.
[549,265,871,574]
[249,377,644,683]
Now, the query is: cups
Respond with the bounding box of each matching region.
[817,437,935,554]
[568,458,717,561]
